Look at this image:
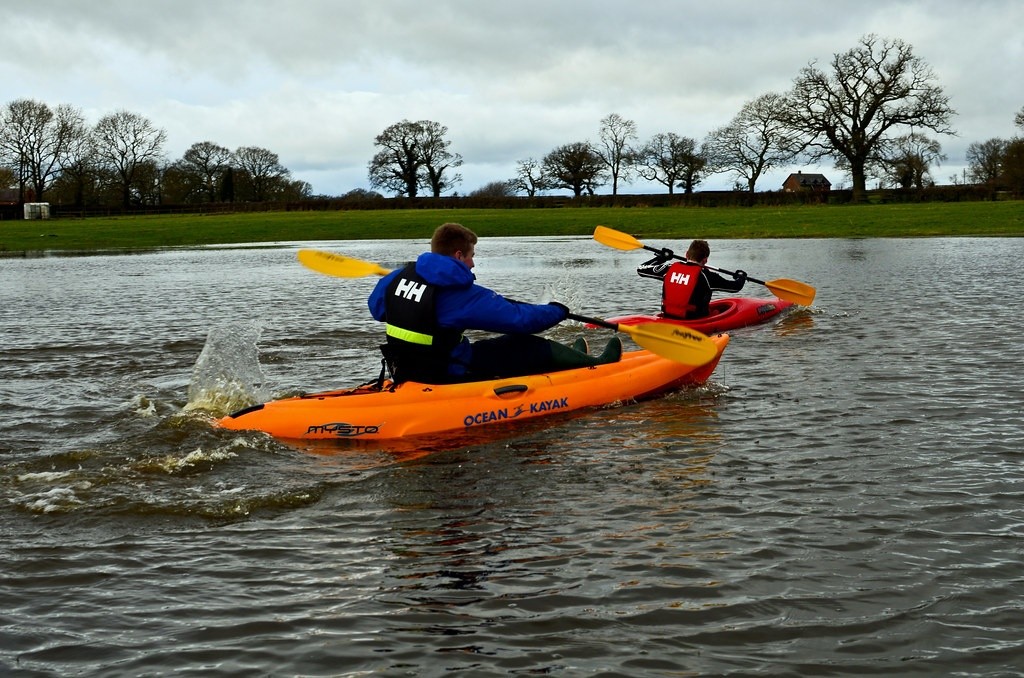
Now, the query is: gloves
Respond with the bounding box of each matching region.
[659,248,674,261]
[733,270,747,279]
[549,302,569,320]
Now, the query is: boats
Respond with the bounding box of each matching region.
[218,332,731,450]
[586,295,794,350]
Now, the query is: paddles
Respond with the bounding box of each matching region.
[298,248,718,367]
[593,225,816,307]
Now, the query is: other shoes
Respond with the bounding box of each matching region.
[574,337,589,354]
[591,337,623,364]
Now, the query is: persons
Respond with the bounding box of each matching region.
[637,240,747,320]
[368,224,622,386]
